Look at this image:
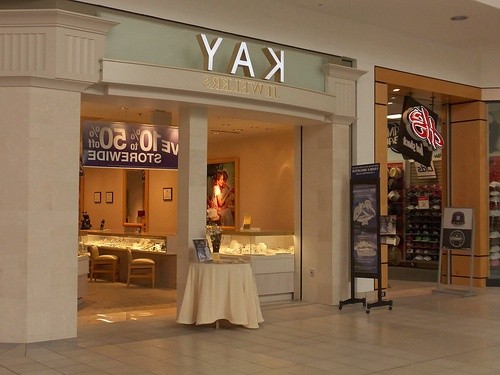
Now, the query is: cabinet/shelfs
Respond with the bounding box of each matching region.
[387,166,442,269]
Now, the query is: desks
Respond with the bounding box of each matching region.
[171,257,265,330]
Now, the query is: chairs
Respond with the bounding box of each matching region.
[88,245,120,283]
[126,247,156,288]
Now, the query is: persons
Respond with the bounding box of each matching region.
[210,171,233,226]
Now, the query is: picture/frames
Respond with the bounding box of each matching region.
[193,239,213,262]
[207,157,240,230]
